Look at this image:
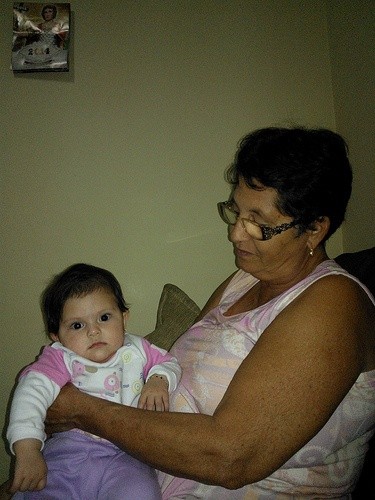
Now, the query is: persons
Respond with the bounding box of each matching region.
[40,126,375,499]
[5,262,185,499]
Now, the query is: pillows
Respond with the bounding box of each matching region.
[142,280,201,355]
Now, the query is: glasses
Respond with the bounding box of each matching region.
[217,199,319,241]
